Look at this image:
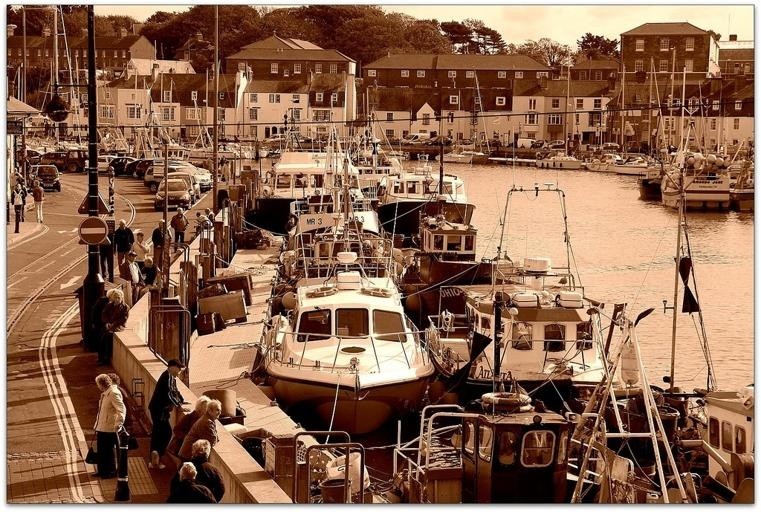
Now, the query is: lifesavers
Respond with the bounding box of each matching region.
[482,392,534,414]
[361,286,392,297]
[306,286,338,297]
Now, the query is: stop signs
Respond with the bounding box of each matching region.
[80,218,108,244]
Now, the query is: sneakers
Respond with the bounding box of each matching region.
[149,462,166,469]
[152,450,159,465]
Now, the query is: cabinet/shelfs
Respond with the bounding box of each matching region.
[265,438,296,500]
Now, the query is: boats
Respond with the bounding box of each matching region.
[251,146,755,504]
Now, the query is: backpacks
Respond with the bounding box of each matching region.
[13,190,23,205]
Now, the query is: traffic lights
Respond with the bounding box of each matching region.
[284,114,288,133]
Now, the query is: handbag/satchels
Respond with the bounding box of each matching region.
[127,436,139,450]
[85,432,97,464]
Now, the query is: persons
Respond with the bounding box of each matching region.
[108,373,131,464]
[171,207,189,253]
[93,289,115,360]
[91,374,126,479]
[148,360,191,470]
[167,395,211,466]
[218,155,229,185]
[194,212,213,237]
[167,462,217,503]
[178,400,222,470]
[178,440,225,503]
[205,208,215,224]
[152,219,163,266]
[91,220,158,302]
[11,168,27,222]
[31,180,45,223]
[100,290,130,364]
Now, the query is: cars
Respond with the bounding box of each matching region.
[260,131,455,142]
[460,136,679,151]
[24,142,211,212]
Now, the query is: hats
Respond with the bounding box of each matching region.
[168,360,185,368]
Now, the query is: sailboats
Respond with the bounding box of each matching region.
[537,45,754,213]
[51,5,252,160]
